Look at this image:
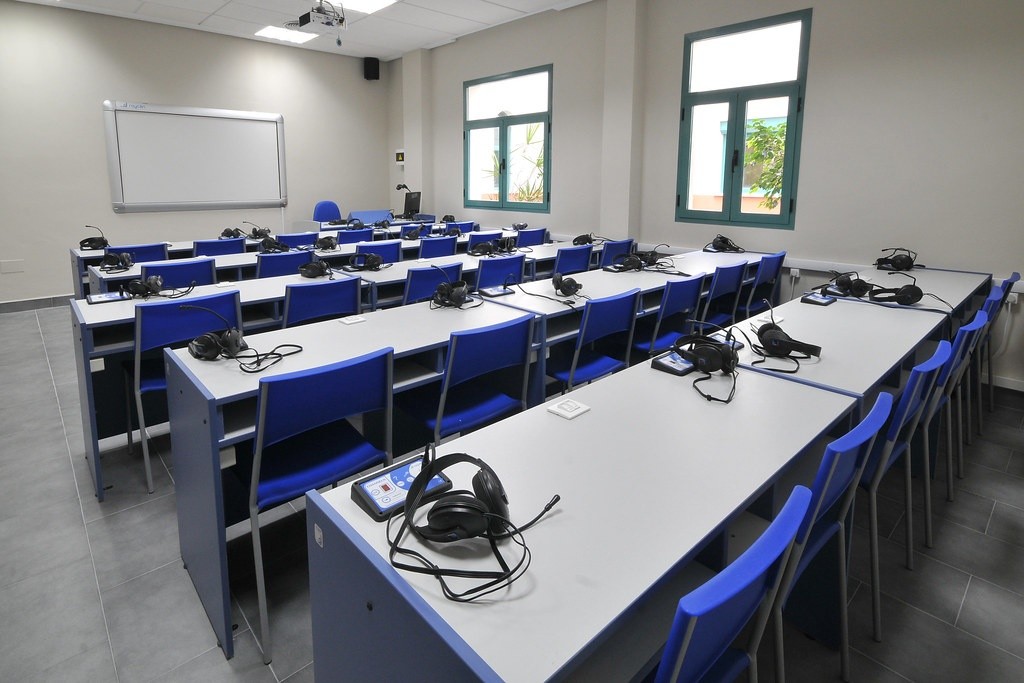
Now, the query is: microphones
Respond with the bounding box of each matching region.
[131,253,136,266]
[243,221,260,229]
[178,304,232,333]
[379,264,393,269]
[685,319,736,350]
[653,244,670,252]
[598,240,605,245]
[502,228,511,231]
[829,271,859,282]
[85,225,103,236]
[888,272,916,286]
[479,494,561,539]
[762,298,775,325]
[881,248,916,256]
[431,264,451,284]
[168,281,196,299]
[389,209,395,214]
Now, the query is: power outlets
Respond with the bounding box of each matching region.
[1004,292,1018,305]
[789,269,801,278]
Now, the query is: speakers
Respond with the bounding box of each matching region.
[364,57,380,80]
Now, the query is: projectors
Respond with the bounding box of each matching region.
[299,12,346,33]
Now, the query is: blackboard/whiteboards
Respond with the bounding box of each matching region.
[102,100,288,213]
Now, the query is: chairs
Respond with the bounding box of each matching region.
[281,277,361,328]
[694,261,747,338]
[140,259,218,290]
[445,221,474,233]
[220,346,393,666]
[255,250,315,279]
[402,262,463,306]
[544,289,642,396]
[312,201,342,222]
[733,250,787,322]
[630,274,707,366]
[355,242,404,265]
[552,245,594,278]
[276,233,320,249]
[650,271,1022,683]
[418,236,458,259]
[392,313,536,448]
[472,254,526,292]
[597,238,634,268]
[117,291,244,495]
[193,237,246,258]
[400,226,433,238]
[336,229,374,245]
[517,228,547,247]
[105,243,169,264]
[467,232,503,252]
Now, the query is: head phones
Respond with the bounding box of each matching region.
[404,454,510,543]
[79,213,528,361]
[551,234,924,374]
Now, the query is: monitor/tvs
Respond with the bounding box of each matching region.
[404,192,421,218]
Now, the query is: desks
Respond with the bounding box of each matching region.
[69,216,994,683]
